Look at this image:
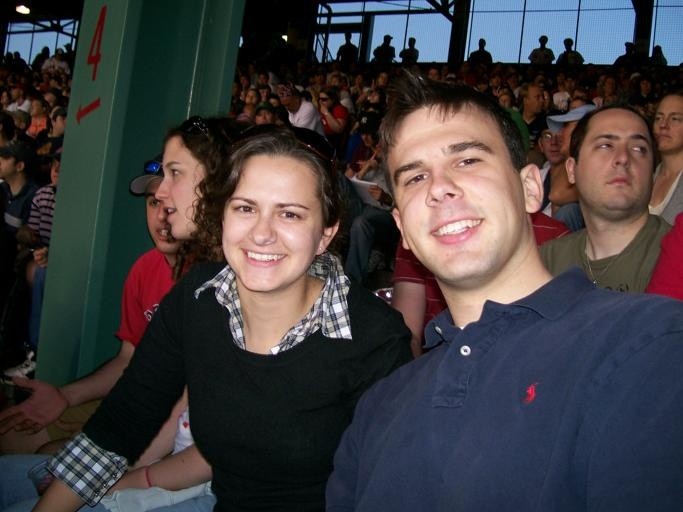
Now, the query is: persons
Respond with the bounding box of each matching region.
[0,30,681,508]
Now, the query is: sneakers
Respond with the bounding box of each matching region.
[0,349,36,386]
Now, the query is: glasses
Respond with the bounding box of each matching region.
[541,133,551,142]
[319,97,329,101]
[144,160,163,175]
[180,116,209,137]
[231,123,339,217]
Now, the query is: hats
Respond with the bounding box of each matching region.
[129,155,164,197]
[355,111,380,131]
[545,104,596,131]
[254,101,274,115]
